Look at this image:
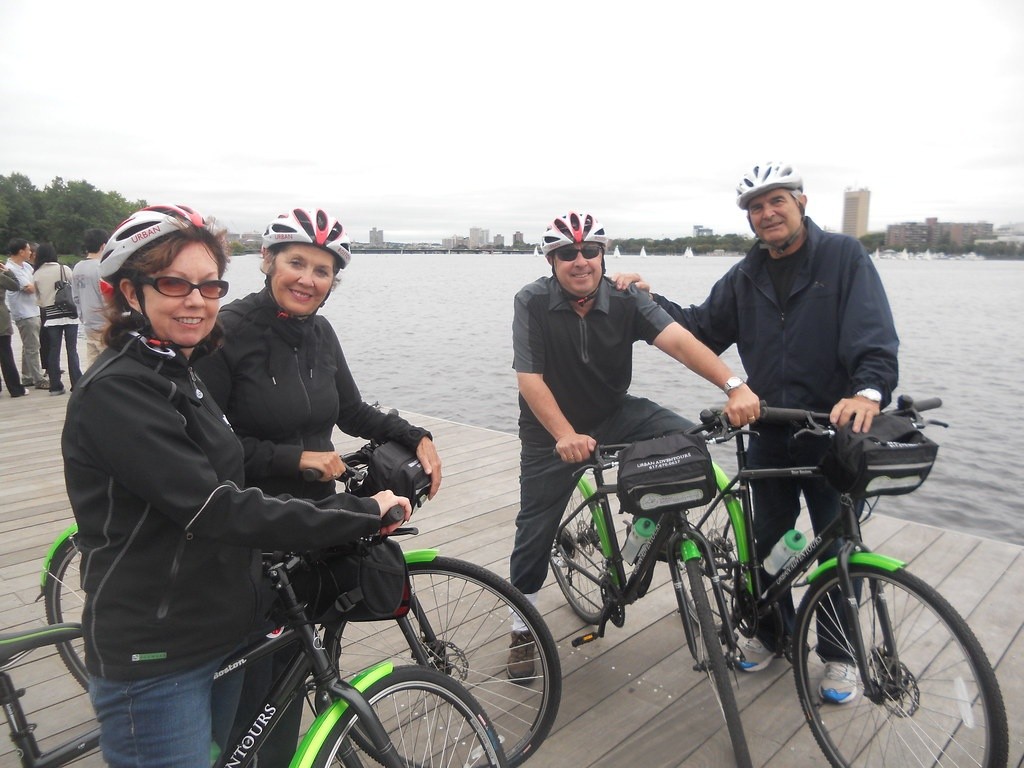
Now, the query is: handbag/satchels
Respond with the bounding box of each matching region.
[54,264,78,319]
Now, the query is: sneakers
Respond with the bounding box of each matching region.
[10,388,29,397]
[656,534,687,563]
[819,661,859,704]
[506,630,537,686]
[735,634,790,673]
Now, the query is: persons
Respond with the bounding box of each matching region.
[0,238,84,397]
[611,162,900,702]
[72,229,108,366]
[61,205,412,768]
[192,207,441,768]
[504,210,760,686]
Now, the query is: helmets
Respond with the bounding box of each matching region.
[540,210,609,257]
[736,157,804,210]
[98,204,226,314]
[262,204,352,270]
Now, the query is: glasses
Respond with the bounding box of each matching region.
[552,245,603,262]
[133,272,229,299]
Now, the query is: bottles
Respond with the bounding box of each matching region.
[763,529,806,576]
[619,518,655,566]
[210,737,221,768]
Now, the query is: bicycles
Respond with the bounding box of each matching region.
[549,398,1009,768]
[0,408,563,768]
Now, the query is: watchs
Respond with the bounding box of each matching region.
[724,376,745,394]
[854,388,881,404]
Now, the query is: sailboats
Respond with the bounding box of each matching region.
[533,246,540,257]
[684,247,694,260]
[613,245,620,257]
[640,245,647,258]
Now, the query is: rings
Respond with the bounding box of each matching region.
[568,455,574,459]
[748,415,754,419]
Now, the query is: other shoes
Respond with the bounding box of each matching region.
[50,386,66,396]
[34,379,50,389]
[21,377,34,386]
